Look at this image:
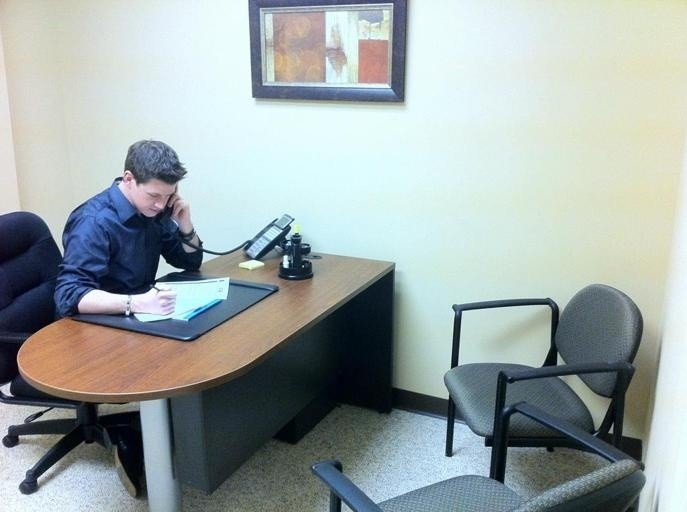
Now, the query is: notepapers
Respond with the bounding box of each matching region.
[238,260,265,271]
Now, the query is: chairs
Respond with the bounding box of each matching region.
[1,210,149,497]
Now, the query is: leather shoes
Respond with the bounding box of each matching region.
[102,424,145,499]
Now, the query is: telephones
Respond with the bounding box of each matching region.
[156,193,175,226]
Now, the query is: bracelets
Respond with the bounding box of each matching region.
[179,229,196,241]
[125,294,133,316]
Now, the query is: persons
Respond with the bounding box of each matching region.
[47,139,204,499]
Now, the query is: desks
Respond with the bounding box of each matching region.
[10,248,398,512]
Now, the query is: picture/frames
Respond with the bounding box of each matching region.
[245,0,408,103]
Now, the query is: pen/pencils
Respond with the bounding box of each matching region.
[150,284,160,291]
